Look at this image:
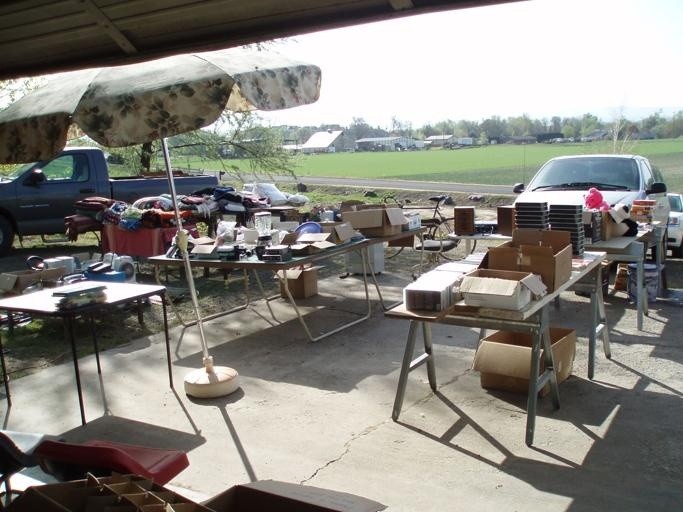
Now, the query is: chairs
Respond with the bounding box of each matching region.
[0,431,189,506]
[411,219,458,264]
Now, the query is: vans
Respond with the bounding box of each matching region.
[506,149,669,246]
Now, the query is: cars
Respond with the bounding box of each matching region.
[661,190,683,256]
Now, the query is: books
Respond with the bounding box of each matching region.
[630,199,656,224]
[51,283,107,297]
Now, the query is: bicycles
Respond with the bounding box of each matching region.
[370,192,480,260]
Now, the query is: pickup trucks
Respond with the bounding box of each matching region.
[0,141,220,248]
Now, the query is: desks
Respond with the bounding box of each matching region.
[148,225,428,342]
[385,251,609,447]
[0,280,173,426]
[584,221,662,330]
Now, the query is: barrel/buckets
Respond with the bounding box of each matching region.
[627,262,664,302]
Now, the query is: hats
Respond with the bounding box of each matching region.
[608,204,630,223]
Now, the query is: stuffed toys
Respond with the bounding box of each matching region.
[583,187,609,211]
[608,203,638,236]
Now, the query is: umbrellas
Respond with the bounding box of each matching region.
[0,53,322,399]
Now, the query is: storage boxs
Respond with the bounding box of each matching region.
[192,200,420,259]
[472,327,575,396]
[459,228,572,311]
[274,263,325,298]
[584,208,623,240]
[0,256,74,291]
[85,270,125,282]
